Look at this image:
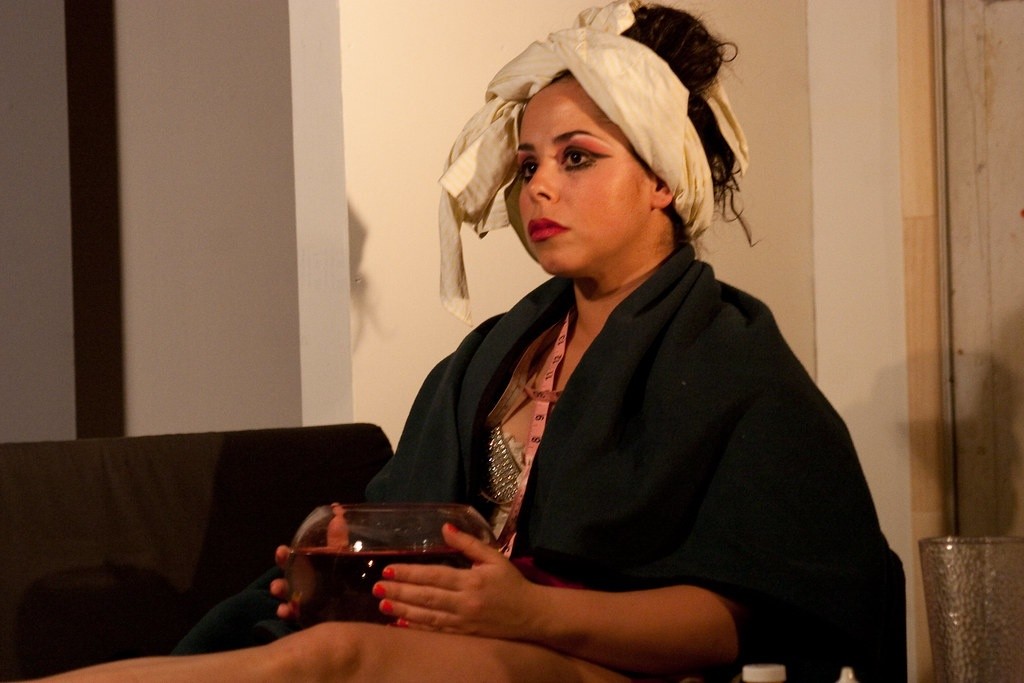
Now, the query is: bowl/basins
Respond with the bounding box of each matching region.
[288,504,498,625]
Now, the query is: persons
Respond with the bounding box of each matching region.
[29,6,884,683]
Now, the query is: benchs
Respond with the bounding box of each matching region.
[1,422,396,683]
